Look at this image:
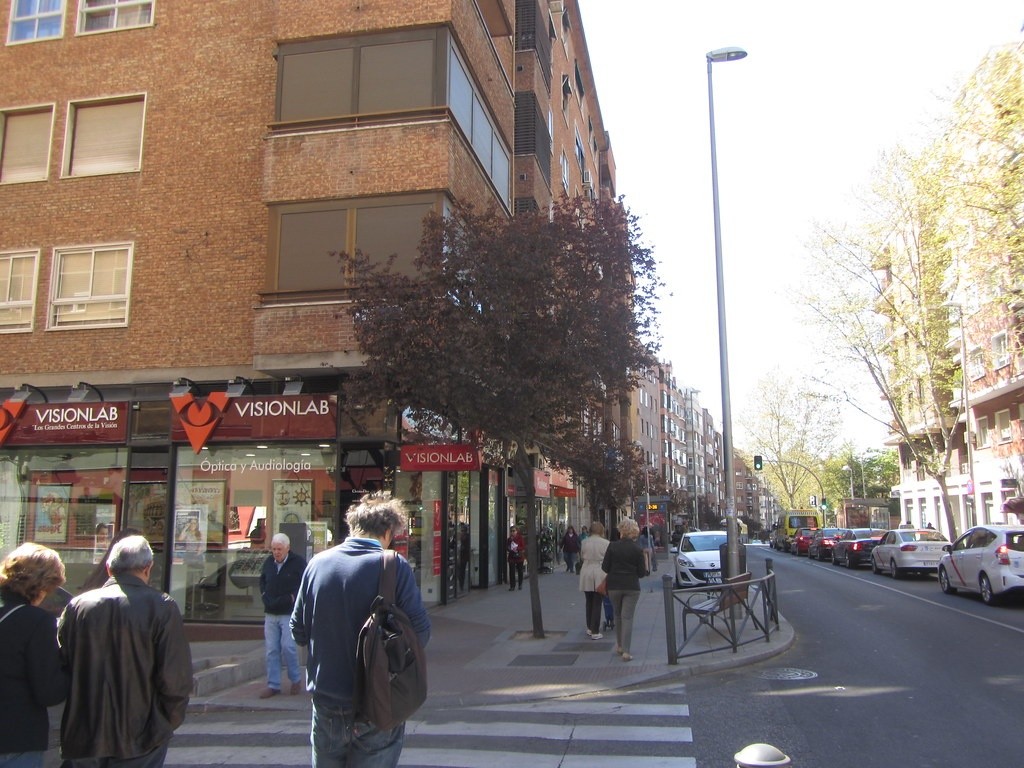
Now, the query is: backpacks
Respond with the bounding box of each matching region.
[354,548,427,730]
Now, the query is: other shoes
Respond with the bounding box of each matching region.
[623,652,632,662]
[587,629,592,636]
[617,647,623,655]
[570,570,575,573]
[291,682,300,695]
[566,566,569,572]
[509,587,515,591]
[259,688,279,699]
[591,633,603,639]
[519,585,522,589]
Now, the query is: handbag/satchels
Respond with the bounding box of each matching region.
[595,575,608,597]
[575,561,582,574]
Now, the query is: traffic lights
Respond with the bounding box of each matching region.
[754,455,763,471]
[822,498,826,510]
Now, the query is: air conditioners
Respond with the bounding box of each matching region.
[583,171,593,187]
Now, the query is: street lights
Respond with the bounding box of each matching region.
[706,45,750,619]
[843,466,856,508]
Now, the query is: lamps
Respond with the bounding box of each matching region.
[224,376,255,397]
[168,377,202,397]
[9,383,48,403]
[67,382,105,402]
[283,375,313,395]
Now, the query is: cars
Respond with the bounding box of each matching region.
[807,528,848,562]
[830,529,889,570]
[792,527,819,556]
[937,524,1024,604]
[870,528,954,580]
[670,531,727,589]
[767,523,778,548]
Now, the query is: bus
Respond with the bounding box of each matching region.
[772,509,821,551]
[720,518,749,545]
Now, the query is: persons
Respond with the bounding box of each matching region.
[579,519,645,661]
[640,527,655,575]
[561,526,578,572]
[180,519,203,556]
[259,534,308,697]
[81,529,141,593]
[926,523,934,529]
[58,535,192,768]
[677,520,689,539]
[0,541,66,768]
[290,493,430,768]
[506,526,525,590]
